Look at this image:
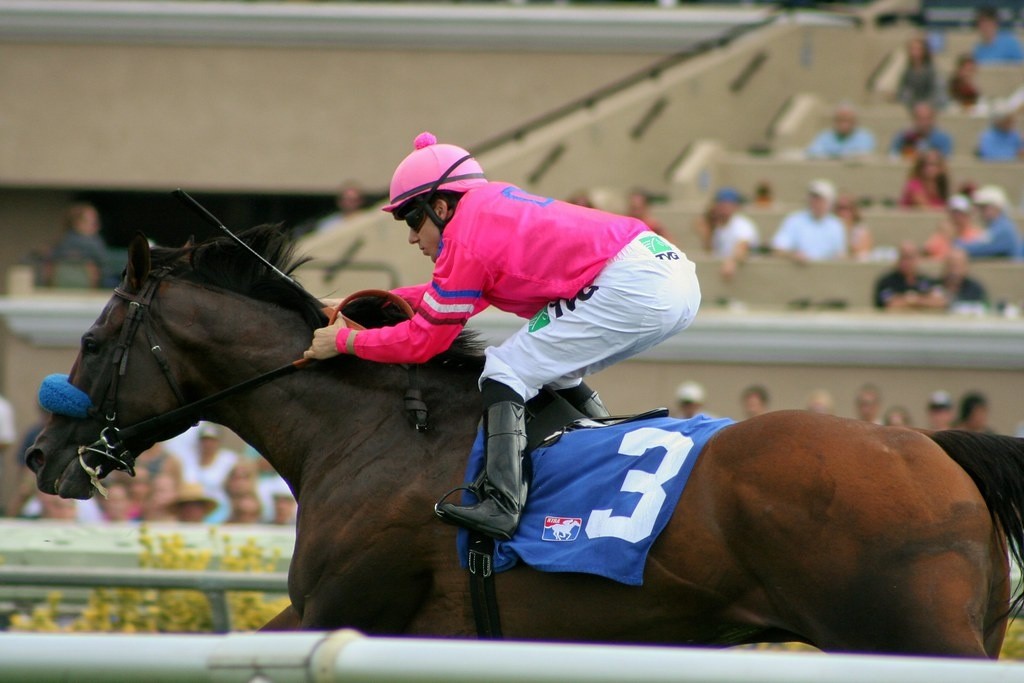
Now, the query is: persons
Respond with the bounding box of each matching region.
[0,393,298,529]
[51,199,112,285]
[570,10,1024,433]
[334,185,363,220]
[303,129,701,539]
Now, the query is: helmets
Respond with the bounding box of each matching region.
[382,130,490,221]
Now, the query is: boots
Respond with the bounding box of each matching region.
[439,401,530,543]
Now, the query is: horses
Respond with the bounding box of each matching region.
[16,232,1024,658]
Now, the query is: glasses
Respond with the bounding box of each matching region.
[403,206,435,233]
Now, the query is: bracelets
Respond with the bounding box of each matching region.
[336,328,355,354]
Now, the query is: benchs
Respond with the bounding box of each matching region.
[655,48,1024,309]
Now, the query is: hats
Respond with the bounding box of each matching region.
[675,381,706,406]
[714,185,745,205]
[946,193,972,214]
[972,185,1007,211]
[806,176,839,203]
